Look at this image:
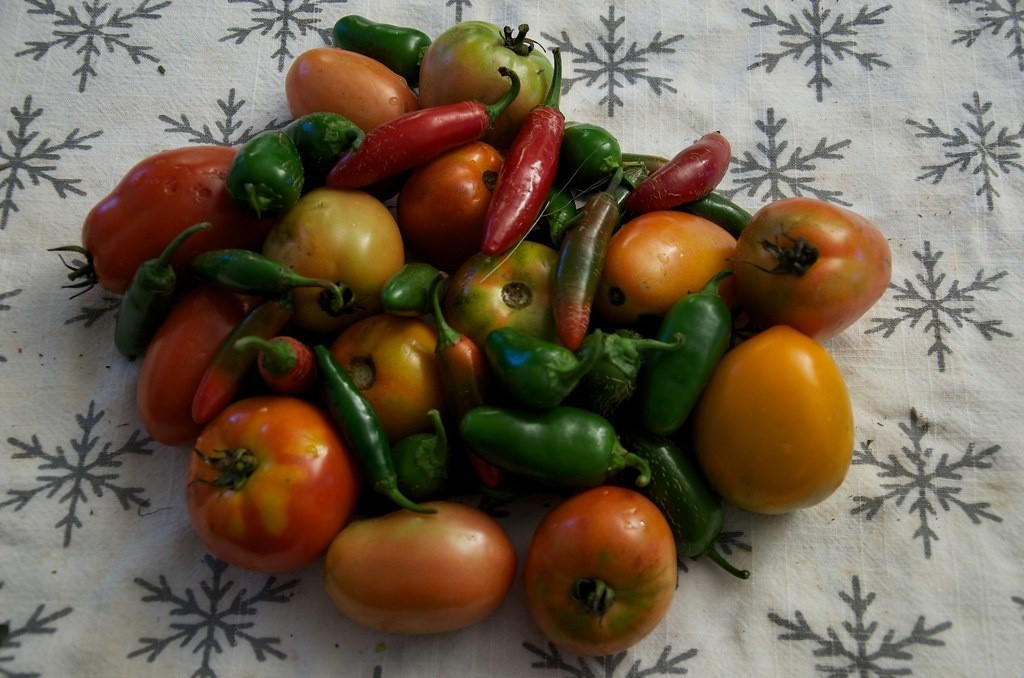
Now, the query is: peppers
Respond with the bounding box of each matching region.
[114,16,753,580]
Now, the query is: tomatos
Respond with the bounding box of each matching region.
[724,197,894,340]
[692,324,855,515]
[417,21,554,142]
[285,47,420,133]
[79,144,677,656]
[590,212,737,329]
